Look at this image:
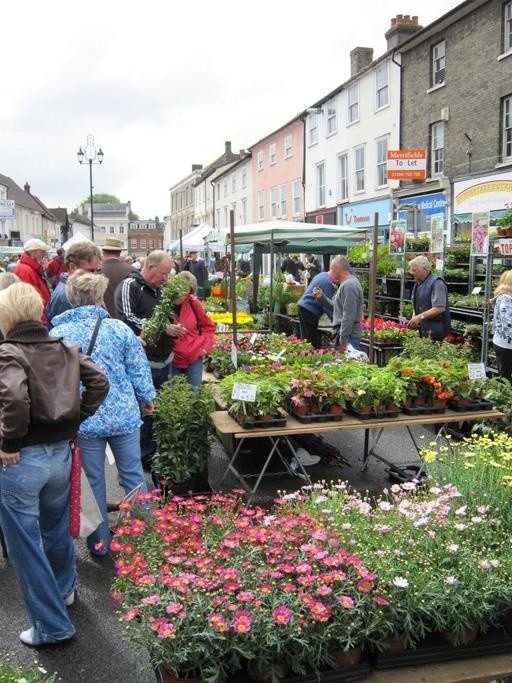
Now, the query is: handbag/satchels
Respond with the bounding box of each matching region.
[80,466,104,538]
[70,432,80,538]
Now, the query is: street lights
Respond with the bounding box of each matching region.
[76,134,104,243]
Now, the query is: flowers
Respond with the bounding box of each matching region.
[98,428,512,683]
[202,310,504,427]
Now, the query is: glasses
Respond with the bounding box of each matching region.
[78,268,102,272]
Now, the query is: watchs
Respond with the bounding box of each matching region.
[418,313,425,321]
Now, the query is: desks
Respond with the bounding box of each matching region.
[210,410,505,503]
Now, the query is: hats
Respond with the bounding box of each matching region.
[24,239,50,252]
[102,238,127,251]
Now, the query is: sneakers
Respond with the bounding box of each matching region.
[20,626,77,647]
[64,590,74,606]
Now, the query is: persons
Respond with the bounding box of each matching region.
[0,281,111,647]
[0,252,23,274]
[280,253,295,276]
[172,250,208,300]
[291,255,306,282]
[406,255,452,345]
[207,253,253,278]
[303,253,322,287]
[296,267,351,350]
[47,267,156,558]
[12,237,52,331]
[99,236,141,318]
[488,268,512,388]
[312,254,364,355]
[45,240,104,332]
[168,270,216,386]
[45,246,68,291]
[113,248,187,474]
[118,252,146,272]
